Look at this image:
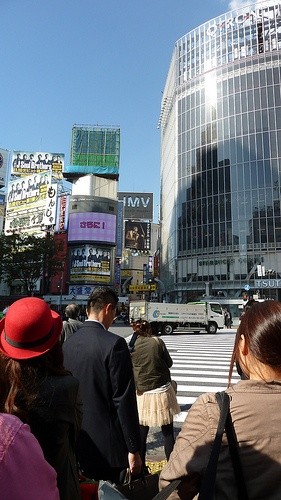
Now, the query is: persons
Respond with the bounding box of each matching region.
[13,153,62,176]
[0,297,88,500]
[124,319,181,463]
[126,225,143,247]
[63,285,144,500]
[72,248,110,269]
[9,174,49,201]
[0,413,61,500]
[158,300,281,500]
[62,302,82,345]
[223,309,233,329]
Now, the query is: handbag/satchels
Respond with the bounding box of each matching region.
[80,474,99,500]
[114,465,162,500]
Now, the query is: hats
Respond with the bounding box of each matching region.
[0,297,62,359]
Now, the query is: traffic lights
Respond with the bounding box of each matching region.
[57,284,61,289]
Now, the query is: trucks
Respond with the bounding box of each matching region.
[129,300,225,336]
